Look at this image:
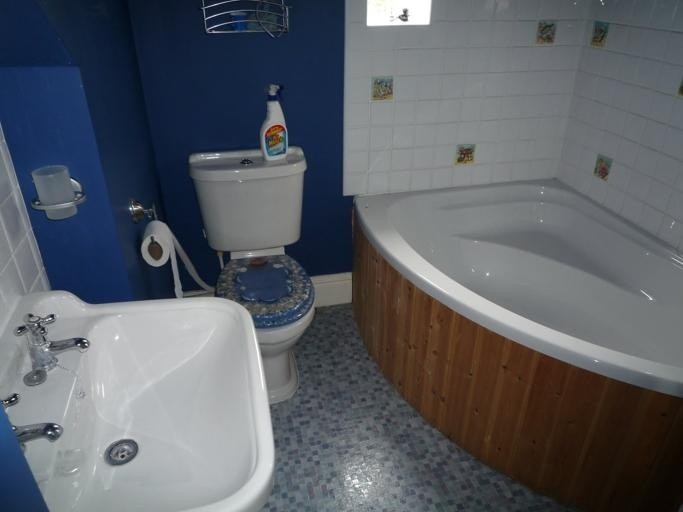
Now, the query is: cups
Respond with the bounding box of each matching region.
[31,164,79,220]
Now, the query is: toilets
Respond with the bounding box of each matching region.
[188,145,315,405]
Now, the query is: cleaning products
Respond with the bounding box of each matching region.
[259,83,289,161]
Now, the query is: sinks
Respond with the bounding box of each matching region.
[0,290,275,511]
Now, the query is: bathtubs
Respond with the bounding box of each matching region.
[353,178,682,512]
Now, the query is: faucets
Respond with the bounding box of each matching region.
[13,313,91,371]
[0,393,63,454]
